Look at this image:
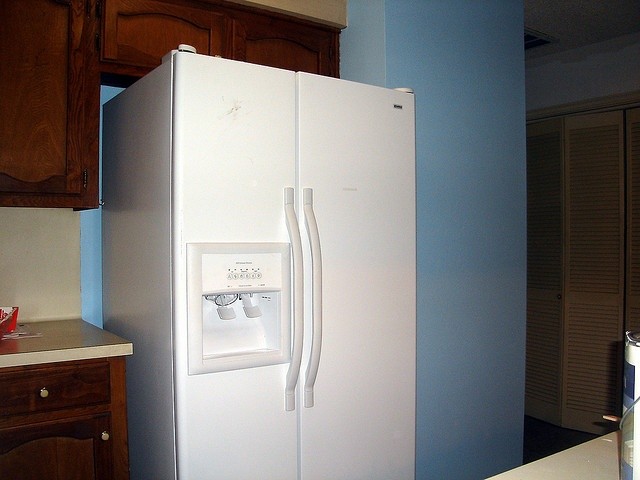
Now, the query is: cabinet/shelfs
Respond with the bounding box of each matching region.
[0,1,97,214]
[100,2,225,84]
[232,11,341,81]
[1,356,135,480]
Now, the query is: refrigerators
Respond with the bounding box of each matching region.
[102,44,416,480]
